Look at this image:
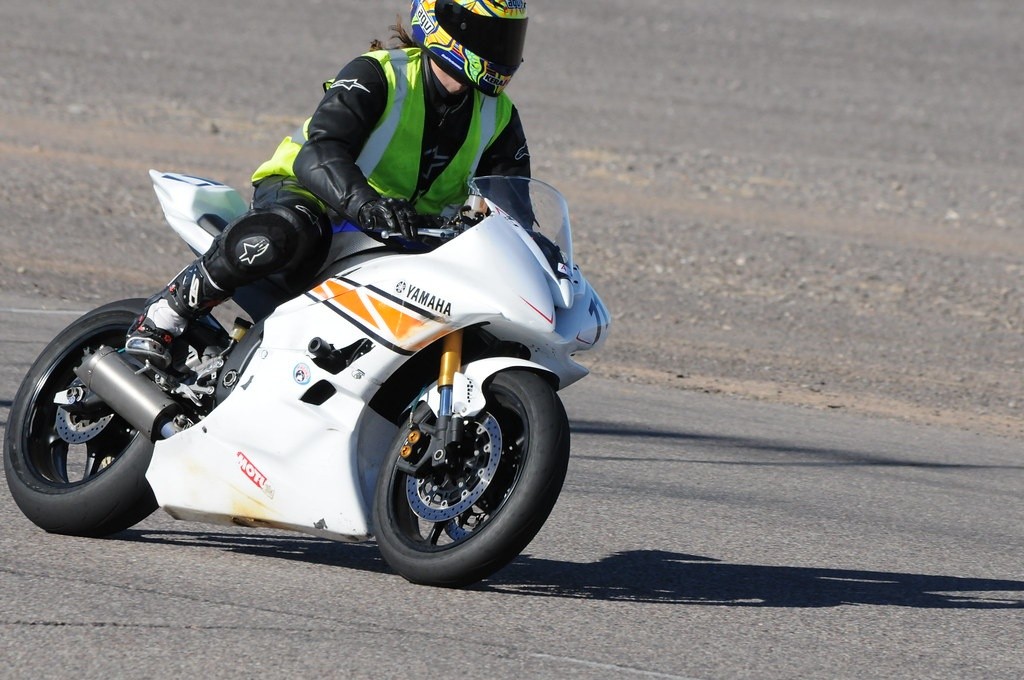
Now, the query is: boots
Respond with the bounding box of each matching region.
[126,256,234,370]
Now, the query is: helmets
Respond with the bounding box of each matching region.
[410,0,530,97]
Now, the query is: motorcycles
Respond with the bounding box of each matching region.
[3,170,612,589]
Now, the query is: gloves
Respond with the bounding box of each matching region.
[358,197,419,240]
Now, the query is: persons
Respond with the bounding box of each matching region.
[128,0,534,363]
